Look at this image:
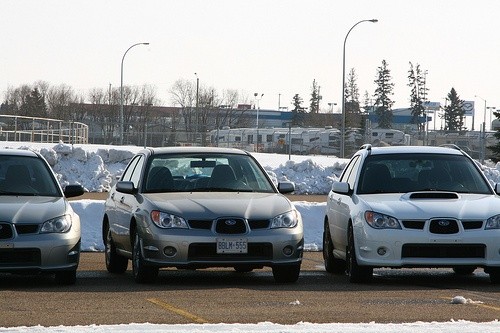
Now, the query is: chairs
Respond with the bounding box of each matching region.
[361,164,400,194]
[207,164,237,188]
[146,166,173,193]
[0,165,39,194]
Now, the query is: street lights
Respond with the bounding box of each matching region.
[121,42,151,145]
[341,19,379,158]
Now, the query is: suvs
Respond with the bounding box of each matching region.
[323,143,500,285]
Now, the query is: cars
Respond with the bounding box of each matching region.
[0,147,82,286]
[103,146,306,285]
